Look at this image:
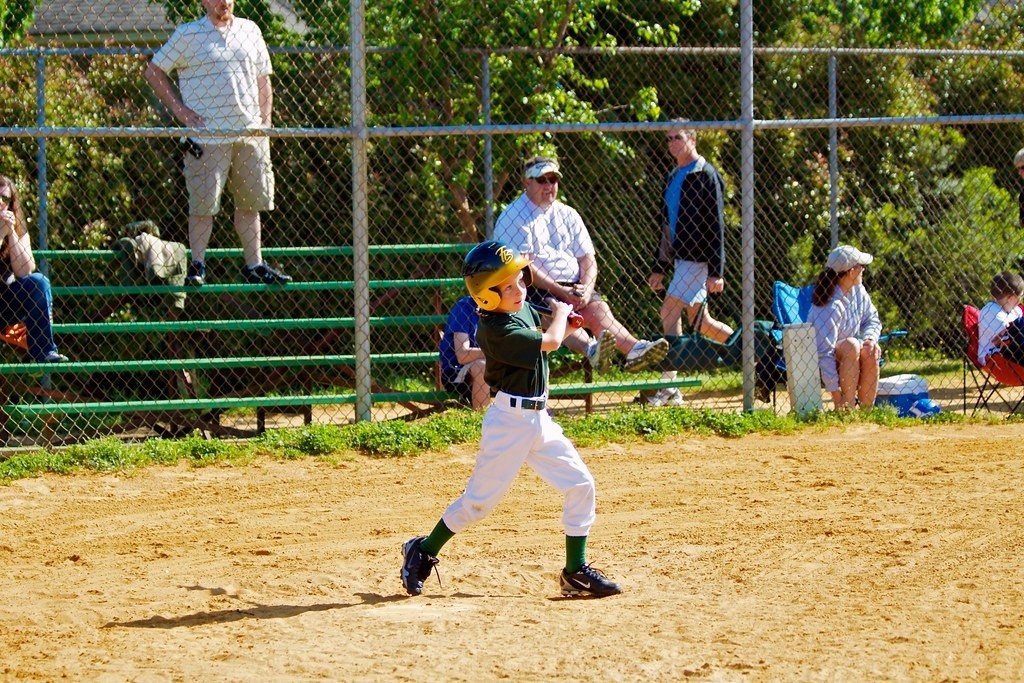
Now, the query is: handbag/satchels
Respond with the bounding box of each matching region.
[652,294,776,403]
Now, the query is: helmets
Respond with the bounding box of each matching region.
[460,239,535,311]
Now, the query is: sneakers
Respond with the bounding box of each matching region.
[187,260,207,285]
[634,387,683,406]
[241,259,293,283]
[586,329,617,375]
[625,337,669,372]
[400,535,442,595]
[560,560,622,597]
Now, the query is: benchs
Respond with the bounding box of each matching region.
[1,238,704,416]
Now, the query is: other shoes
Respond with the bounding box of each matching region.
[24,350,69,361]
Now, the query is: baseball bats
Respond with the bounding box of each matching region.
[530,302,585,329]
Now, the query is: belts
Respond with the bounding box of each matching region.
[511,395,546,410]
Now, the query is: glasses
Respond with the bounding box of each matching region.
[530,175,559,184]
[666,135,683,142]
[1015,164,1024,172]
[1,195,11,203]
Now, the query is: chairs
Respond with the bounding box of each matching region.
[962,304,1024,422]
[774,281,910,410]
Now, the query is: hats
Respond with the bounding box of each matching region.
[826,244,873,271]
[525,161,563,179]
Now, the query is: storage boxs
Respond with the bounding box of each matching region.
[877,371,927,421]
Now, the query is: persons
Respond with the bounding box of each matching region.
[492,156,669,376]
[978,271,1024,365]
[1013,147,1024,228]
[0,174,70,361]
[439,296,498,410]
[634,117,733,406]
[398,239,621,599]
[805,245,883,414]
[144,0,295,285]
